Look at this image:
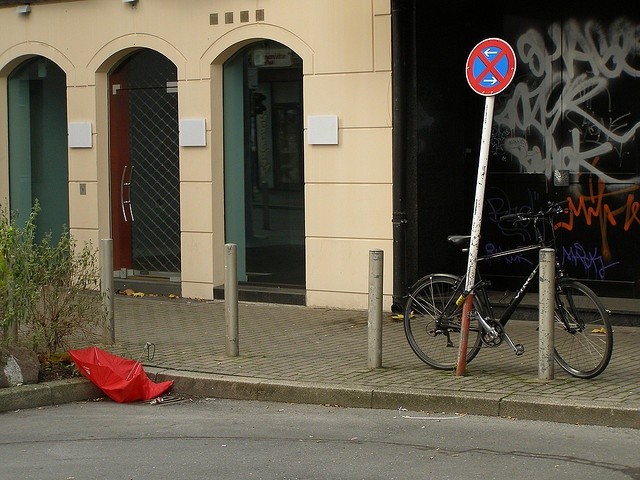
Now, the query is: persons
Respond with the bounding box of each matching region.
[272,144,302,194]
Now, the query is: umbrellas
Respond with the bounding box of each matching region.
[68,342,175,402]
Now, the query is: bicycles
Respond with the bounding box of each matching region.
[404,200,613,379]
